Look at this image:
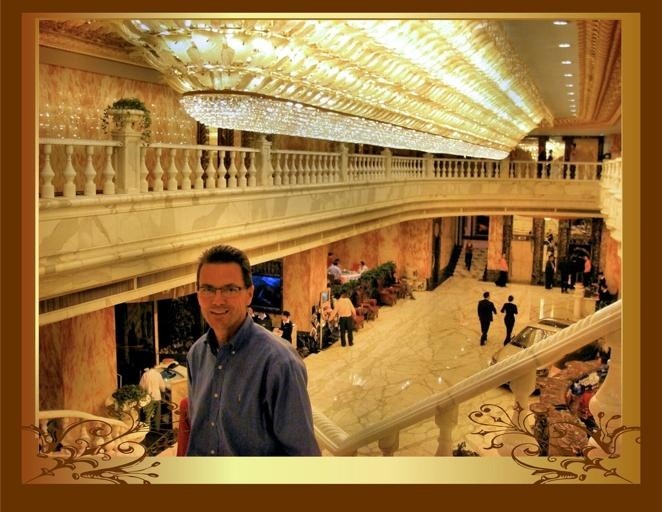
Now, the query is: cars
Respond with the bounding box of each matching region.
[489,317,606,396]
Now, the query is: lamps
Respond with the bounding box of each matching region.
[109,19,556,161]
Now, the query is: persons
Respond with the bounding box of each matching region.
[500,295,518,347]
[185,244,323,457]
[595,271,606,287]
[254,309,272,332]
[494,252,508,287]
[357,260,368,275]
[330,258,341,267]
[246,303,256,321]
[276,309,294,344]
[544,255,556,288]
[570,143,578,179]
[327,290,357,346]
[546,233,554,254]
[138,363,166,433]
[547,148,553,178]
[464,240,473,271]
[309,304,320,343]
[595,284,610,303]
[327,251,335,268]
[328,261,342,285]
[583,255,592,288]
[556,256,571,293]
[477,291,497,346]
[391,270,417,300]
[537,143,546,179]
[569,256,579,289]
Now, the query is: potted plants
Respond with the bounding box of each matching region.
[101,98,153,142]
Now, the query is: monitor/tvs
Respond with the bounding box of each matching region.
[250,272,283,315]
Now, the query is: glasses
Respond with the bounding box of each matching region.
[197,286,246,298]
